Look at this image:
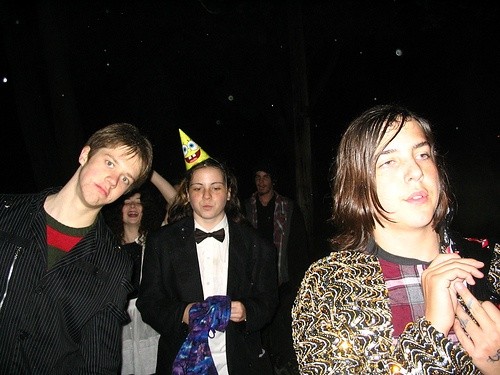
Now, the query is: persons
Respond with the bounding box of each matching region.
[113,129,294,375]
[0,123,153,375]
[290,105,500,375]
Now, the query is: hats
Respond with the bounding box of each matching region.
[179,128,211,172]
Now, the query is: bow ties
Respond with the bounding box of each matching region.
[194,228,225,244]
